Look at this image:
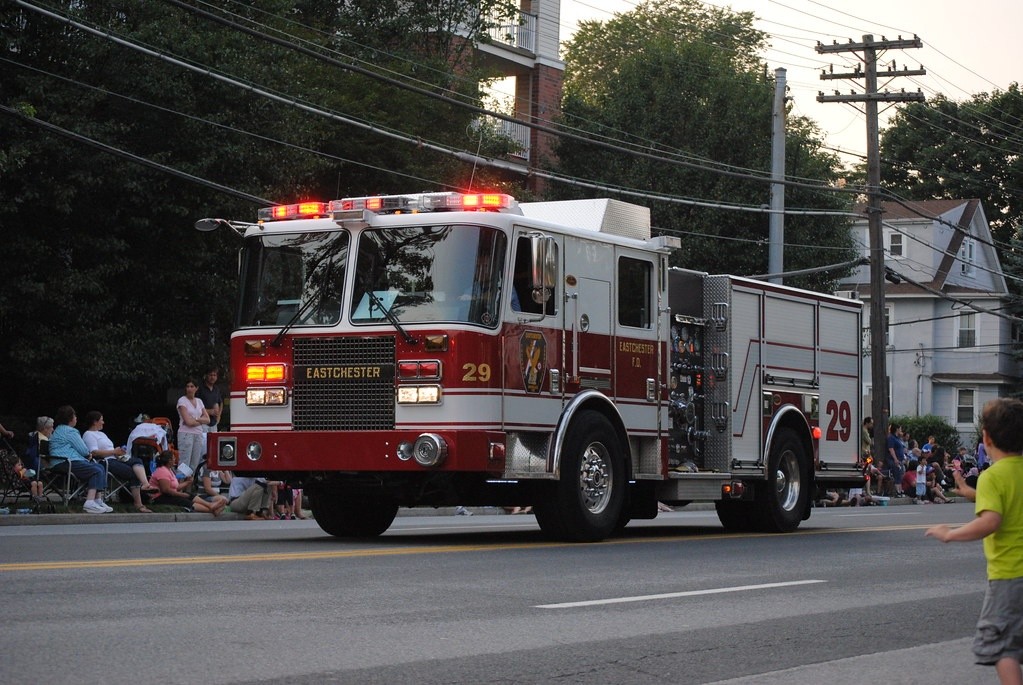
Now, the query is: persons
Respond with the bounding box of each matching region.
[176,381,211,494]
[459,250,521,315]
[812,416,991,509]
[925,398,1023,685]
[195,367,224,466]
[0,405,308,522]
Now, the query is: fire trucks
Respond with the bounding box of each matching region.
[194,188,865,534]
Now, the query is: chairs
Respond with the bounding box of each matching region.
[131,436,161,474]
[92,455,133,503]
[3,435,64,512]
[37,439,97,511]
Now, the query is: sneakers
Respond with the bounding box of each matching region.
[83,501,106,513]
[98,500,113,512]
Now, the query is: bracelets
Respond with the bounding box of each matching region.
[195,417,198,421]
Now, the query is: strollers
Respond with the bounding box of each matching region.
[127,417,174,474]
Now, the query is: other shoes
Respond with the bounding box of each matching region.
[141,484,159,493]
[245,512,264,519]
[924,499,929,504]
[917,499,924,504]
[134,505,152,513]
[262,510,311,521]
